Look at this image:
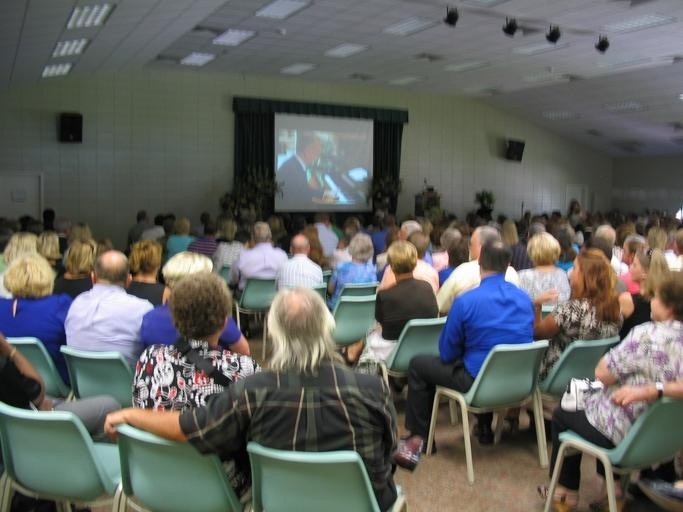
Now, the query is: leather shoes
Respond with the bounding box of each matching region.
[242,328,253,341]
[399,433,439,455]
[472,423,495,448]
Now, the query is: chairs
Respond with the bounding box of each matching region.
[546,396,683,512]
[246,441,408,512]
[332,294,376,364]
[231,276,275,332]
[336,284,378,297]
[0,401,128,511]
[513,336,624,466]
[218,265,229,283]
[60,345,134,412]
[425,339,548,486]
[377,316,447,396]
[4,335,68,408]
[262,286,327,361]
[109,424,252,512]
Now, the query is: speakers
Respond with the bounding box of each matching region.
[506,140,525,162]
[57,112,82,144]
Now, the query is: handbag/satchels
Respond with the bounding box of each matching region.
[561,377,605,413]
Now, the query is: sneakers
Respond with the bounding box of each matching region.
[534,483,579,511]
[588,494,625,512]
[499,417,522,440]
[396,435,424,469]
[335,344,356,367]
[636,478,683,512]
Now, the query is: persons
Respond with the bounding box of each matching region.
[275,128,336,205]
[2,199,683,512]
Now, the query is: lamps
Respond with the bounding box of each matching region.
[594,35,610,54]
[546,25,560,43]
[444,6,460,27]
[502,17,518,37]
